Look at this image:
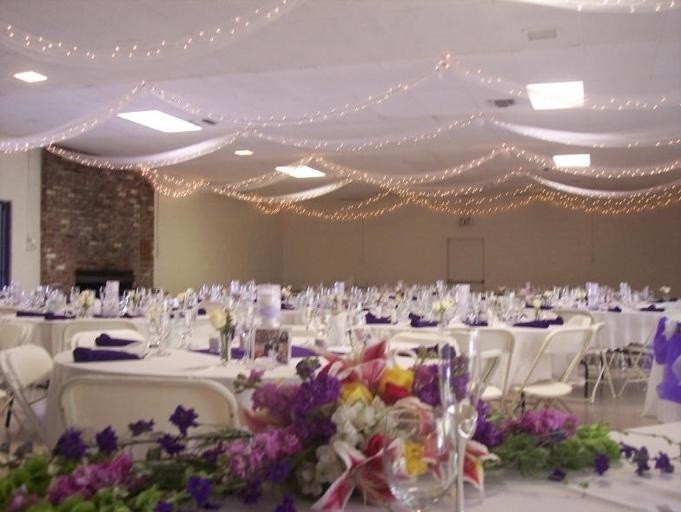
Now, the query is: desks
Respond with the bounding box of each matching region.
[0,298,681,512]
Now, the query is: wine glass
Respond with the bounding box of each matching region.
[0,281,166,317]
[407,278,665,324]
[138,277,406,357]
[381,337,498,510]
